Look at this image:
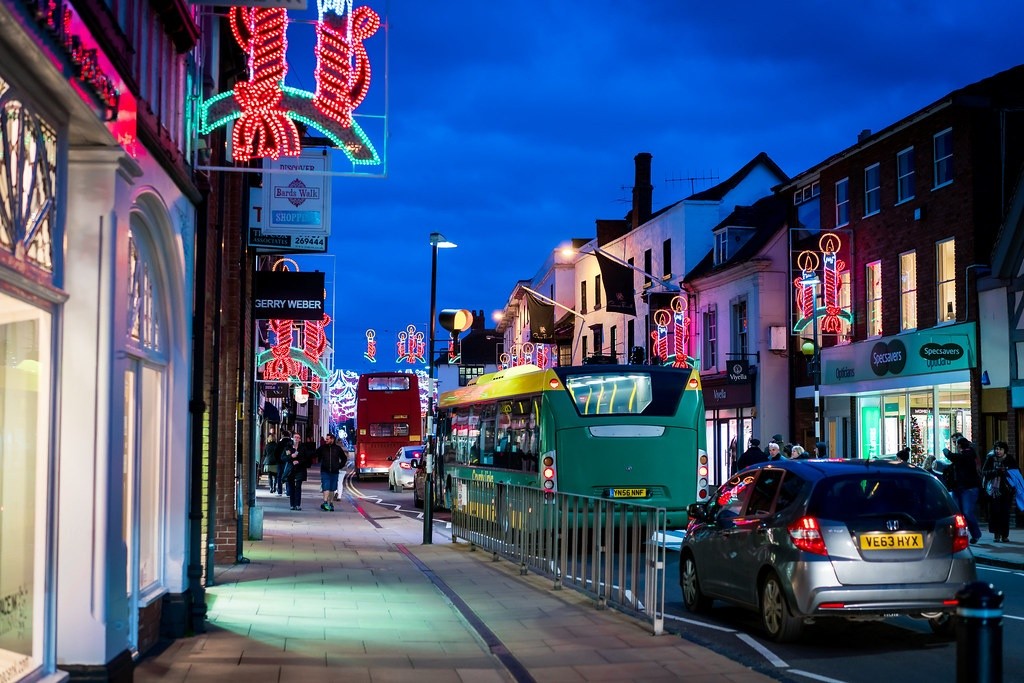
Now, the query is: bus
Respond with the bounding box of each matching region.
[354,373,421,482]
[411,364,708,556]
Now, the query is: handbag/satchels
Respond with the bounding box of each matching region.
[1002,476,1016,496]
[941,468,957,493]
[981,474,1000,501]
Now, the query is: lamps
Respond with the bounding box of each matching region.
[438,308,473,355]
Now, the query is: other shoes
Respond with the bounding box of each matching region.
[970,536,979,544]
[321,503,329,511]
[295,506,302,510]
[329,504,335,511]
[270,487,273,492]
[993,538,1000,542]
[273,486,277,492]
[334,491,338,499]
[1002,537,1010,542]
[290,506,295,510]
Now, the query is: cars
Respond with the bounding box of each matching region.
[679,458,976,645]
[385,446,432,494]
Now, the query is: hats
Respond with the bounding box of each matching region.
[749,439,760,447]
[793,444,806,456]
[771,433,783,442]
[895,447,911,461]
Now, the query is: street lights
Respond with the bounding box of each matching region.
[421,233,474,544]
[798,278,820,442]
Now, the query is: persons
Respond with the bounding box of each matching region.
[897,447,910,462]
[944,433,982,544]
[263,428,316,510]
[737,433,828,470]
[982,441,1019,542]
[318,433,347,511]
